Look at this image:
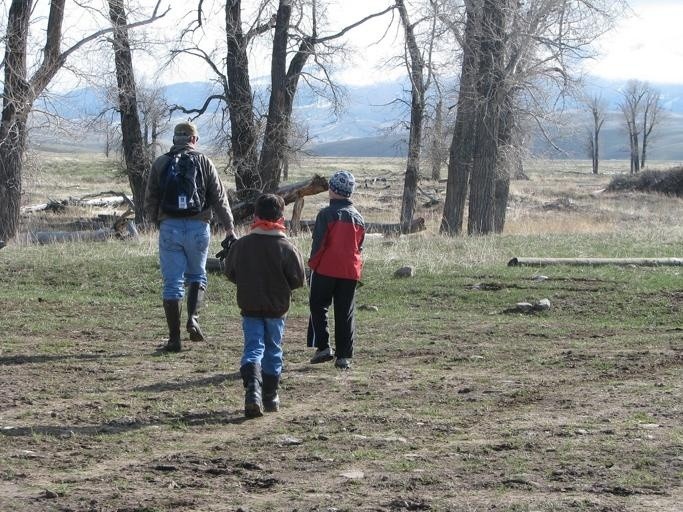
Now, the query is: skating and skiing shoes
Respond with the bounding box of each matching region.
[309,347,334,364]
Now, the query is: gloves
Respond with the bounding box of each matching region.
[214,234,236,262]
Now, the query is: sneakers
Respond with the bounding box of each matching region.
[334,356,350,369]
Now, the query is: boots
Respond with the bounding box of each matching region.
[238,361,264,418]
[160,298,184,353]
[260,367,281,413]
[185,280,207,342]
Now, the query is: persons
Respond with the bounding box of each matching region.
[221,193,305,419]
[306,169,366,370]
[140,123,241,353]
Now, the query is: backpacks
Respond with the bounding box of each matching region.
[160,151,210,217]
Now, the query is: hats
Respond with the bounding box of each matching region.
[327,170,356,198]
[172,121,199,143]
